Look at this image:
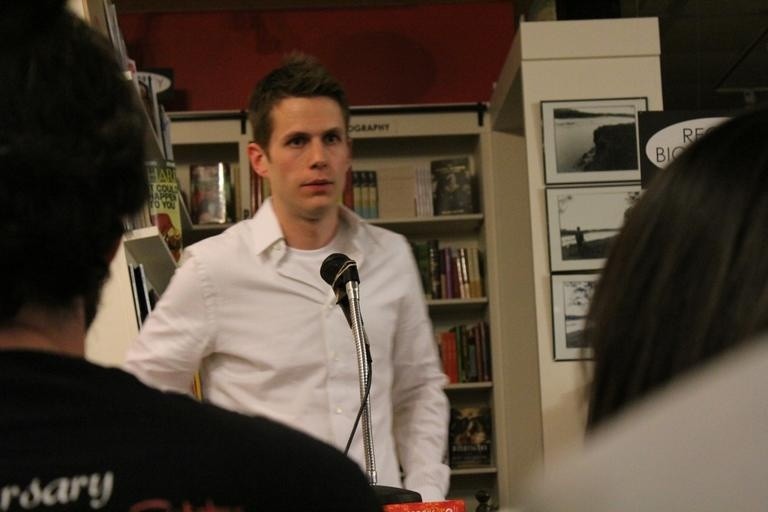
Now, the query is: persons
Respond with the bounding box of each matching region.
[584,106,767,439]
[1,0,382,512]
[123,51,452,503]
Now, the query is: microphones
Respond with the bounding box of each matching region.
[319,252,422,504]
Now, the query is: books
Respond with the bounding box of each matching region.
[340,165,379,218]
[412,240,484,299]
[438,322,492,469]
[248,166,271,217]
[128,159,240,326]
[414,160,474,217]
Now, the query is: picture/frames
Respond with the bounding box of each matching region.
[540,96,649,185]
[543,183,651,271]
[547,271,604,363]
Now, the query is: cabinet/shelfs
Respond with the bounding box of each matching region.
[84,103,508,512]
